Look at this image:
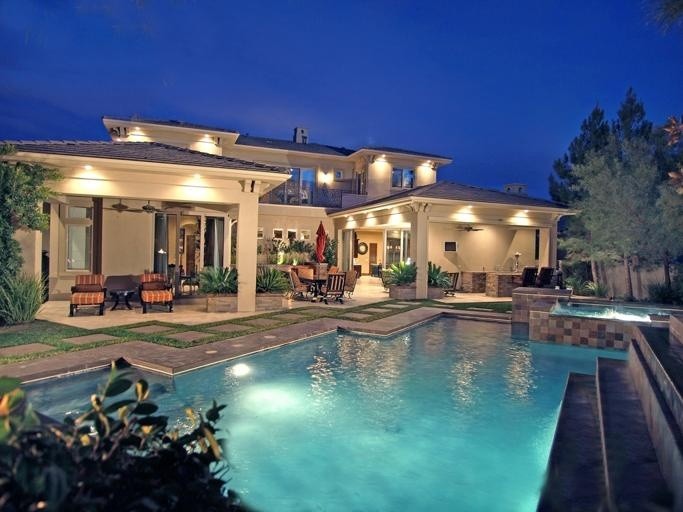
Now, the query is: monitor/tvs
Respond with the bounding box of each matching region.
[444,241,456,251]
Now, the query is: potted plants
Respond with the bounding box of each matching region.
[195,264,291,312]
[381,260,453,301]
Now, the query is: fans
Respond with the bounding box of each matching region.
[454,223,485,232]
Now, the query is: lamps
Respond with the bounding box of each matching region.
[112,199,154,214]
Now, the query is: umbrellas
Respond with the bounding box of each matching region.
[312,221,327,280]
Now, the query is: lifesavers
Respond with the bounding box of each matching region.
[358,242,368,255]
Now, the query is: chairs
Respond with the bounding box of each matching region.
[289,263,359,306]
[67,270,176,318]
[507,263,555,289]
[179,271,199,296]
